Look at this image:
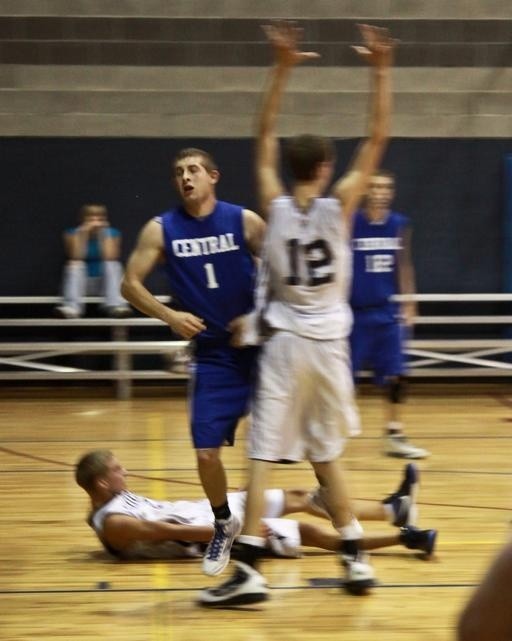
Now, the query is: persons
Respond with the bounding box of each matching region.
[188,20,398,610]
[52,203,133,318]
[350,170,430,461]
[119,148,269,579]
[75,449,437,556]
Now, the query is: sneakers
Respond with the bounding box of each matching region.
[110,306,133,316]
[193,561,270,606]
[383,464,419,528]
[201,513,240,577]
[399,524,436,553]
[56,306,78,319]
[340,549,378,593]
[384,434,431,459]
